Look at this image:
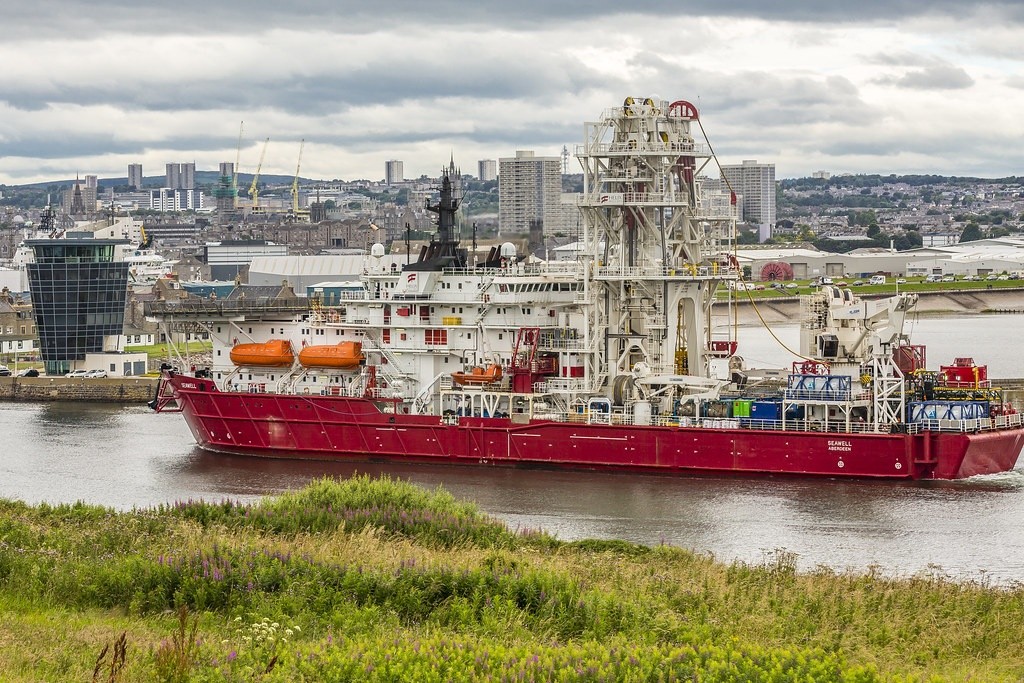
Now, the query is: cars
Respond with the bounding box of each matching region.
[17,369,39,377]
[0,369,12,376]
[64,367,86,378]
[730,272,1024,291]
[84,370,108,378]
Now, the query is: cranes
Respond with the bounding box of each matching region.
[248,137,270,205]
[290,137,306,224]
[231,120,244,208]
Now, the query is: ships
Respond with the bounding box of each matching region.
[148,94,1024,483]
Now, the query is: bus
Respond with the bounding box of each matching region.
[870,274,886,285]
[926,273,943,284]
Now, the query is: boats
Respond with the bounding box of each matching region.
[449,363,503,386]
[298,339,365,370]
[229,338,295,368]
